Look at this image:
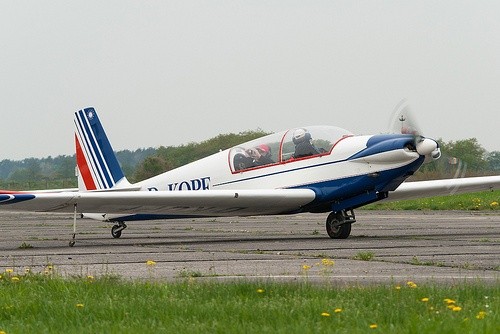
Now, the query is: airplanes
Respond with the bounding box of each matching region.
[0,94,499,248]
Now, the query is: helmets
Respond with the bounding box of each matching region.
[292,128,311,145]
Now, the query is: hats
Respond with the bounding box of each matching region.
[256,144,269,153]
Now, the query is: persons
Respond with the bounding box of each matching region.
[290,128,328,160]
[239,144,275,171]
